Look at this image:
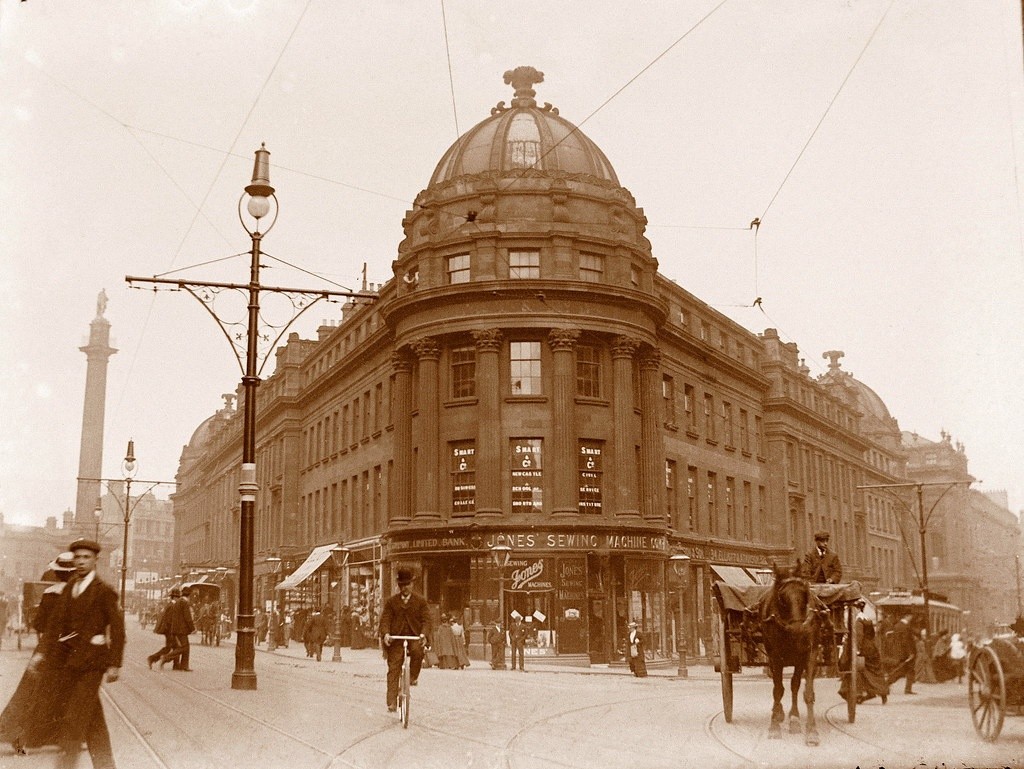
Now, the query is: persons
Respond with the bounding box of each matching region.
[1009,617,1024,656]
[201,592,212,604]
[146,588,195,671]
[508,616,528,671]
[290,599,381,662]
[801,531,843,584]
[253,605,295,649]
[965,636,980,669]
[221,608,232,640]
[913,629,967,684]
[837,599,889,705]
[139,602,150,621]
[378,570,433,712]
[0,538,126,768]
[878,608,894,674]
[434,613,471,670]
[626,622,648,677]
[487,618,506,670]
[886,608,917,695]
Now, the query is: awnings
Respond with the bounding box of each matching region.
[710,564,777,587]
[275,543,339,596]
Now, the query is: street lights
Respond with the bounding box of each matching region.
[126,141,380,690]
[76,495,131,545]
[75,438,181,608]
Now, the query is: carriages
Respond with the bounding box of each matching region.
[714,564,860,746]
[182,582,223,648]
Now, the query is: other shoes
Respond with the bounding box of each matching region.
[410,678,417,685]
[148,656,154,669]
[904,689,917,695]
[173,663,181,670]
[180,665,193,671]
[388,702,398,712]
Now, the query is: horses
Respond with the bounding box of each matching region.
[761,557,822,748]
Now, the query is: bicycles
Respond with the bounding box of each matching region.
[383,635,425,730]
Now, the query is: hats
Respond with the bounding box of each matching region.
[628,622,639,628]
[495,620,501,623]
[396,567,417,583]
[815,531,829,540]
[169,589,180,596]
[50,552,77,571]
[69,540,102,553]
[902,607,914,614]
